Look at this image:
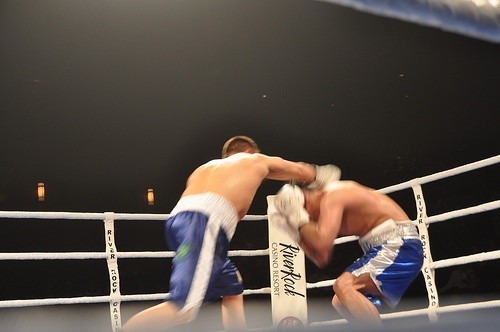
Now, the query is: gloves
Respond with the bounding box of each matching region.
[273,184,309,232]
[306,165,340,191]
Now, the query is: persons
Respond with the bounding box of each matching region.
[276,179,424,332]
[118,136,344,331]
